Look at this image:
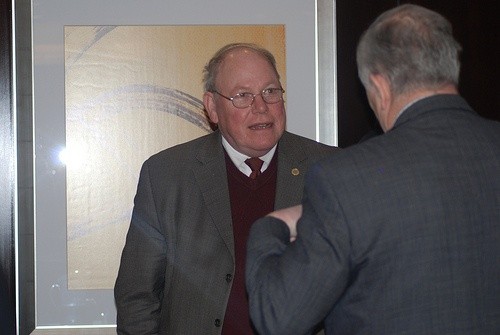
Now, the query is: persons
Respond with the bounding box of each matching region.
[242,3,500,335]
[112,41,345,335]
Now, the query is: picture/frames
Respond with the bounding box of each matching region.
[13,0,340,335]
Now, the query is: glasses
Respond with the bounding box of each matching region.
[210,85,285,108]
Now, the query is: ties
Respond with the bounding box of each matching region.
[244,157,264,179]
